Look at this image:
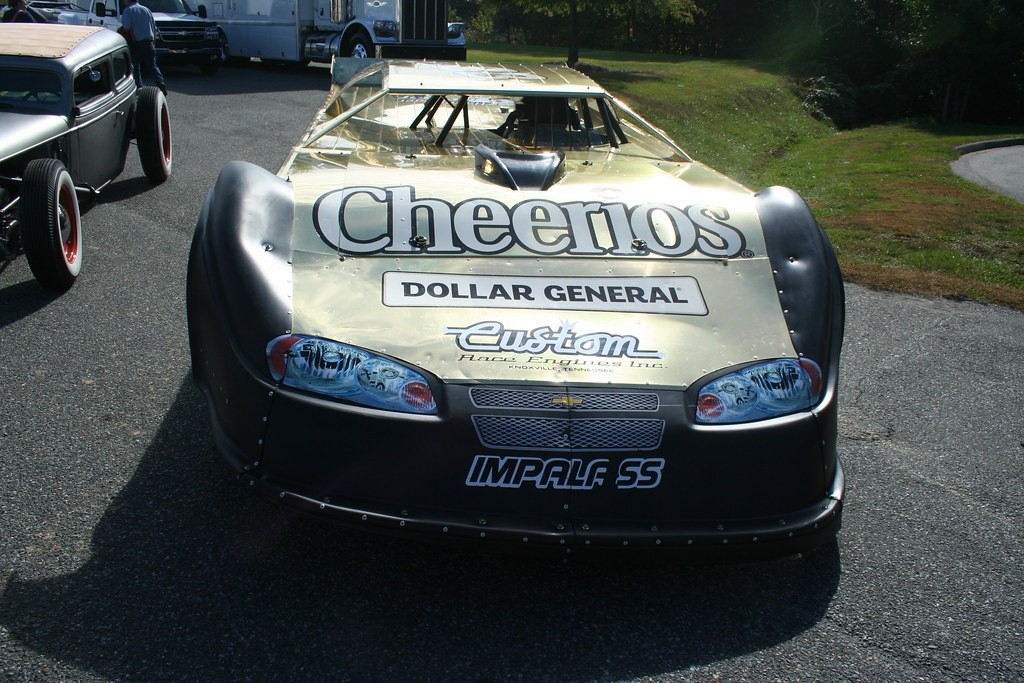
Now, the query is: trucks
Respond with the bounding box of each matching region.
[176,0,469,71]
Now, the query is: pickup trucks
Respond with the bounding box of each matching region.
[0,0,230,77]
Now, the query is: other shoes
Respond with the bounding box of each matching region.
[137,82,143,88]
[156,82,167,97]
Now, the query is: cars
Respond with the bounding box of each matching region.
[0,21,175,288]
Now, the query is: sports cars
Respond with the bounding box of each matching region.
[184,54,849,559]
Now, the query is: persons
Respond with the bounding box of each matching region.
[2,0,36,23]
[503,96,585,146]
[122,0,167,97]
